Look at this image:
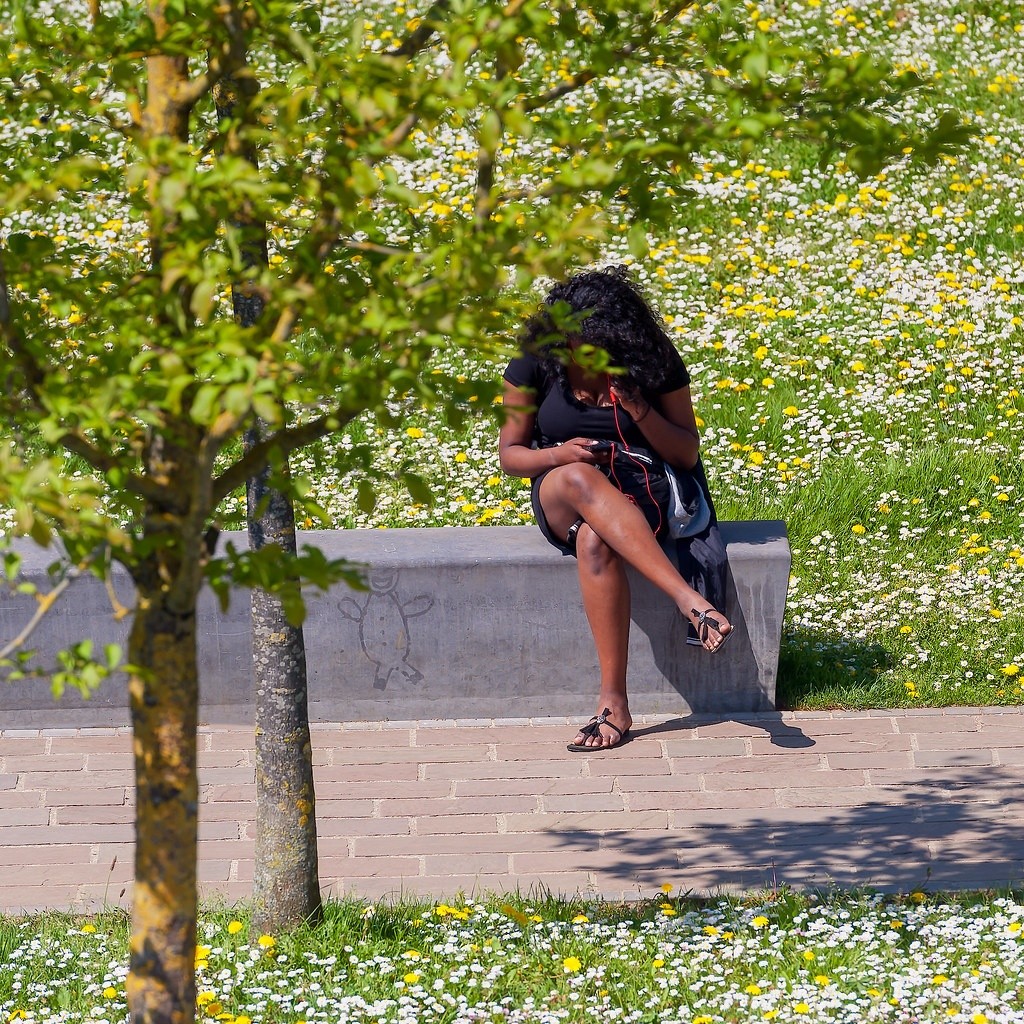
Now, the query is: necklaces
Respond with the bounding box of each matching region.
[571,356,585,368]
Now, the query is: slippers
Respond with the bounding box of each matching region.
[690,608,736,655]
[567,708,630,752]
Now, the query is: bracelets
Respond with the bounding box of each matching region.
[633,402,652,426]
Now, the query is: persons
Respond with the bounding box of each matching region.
[498,275,733,751]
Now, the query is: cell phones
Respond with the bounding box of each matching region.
[583,442,618,452]
